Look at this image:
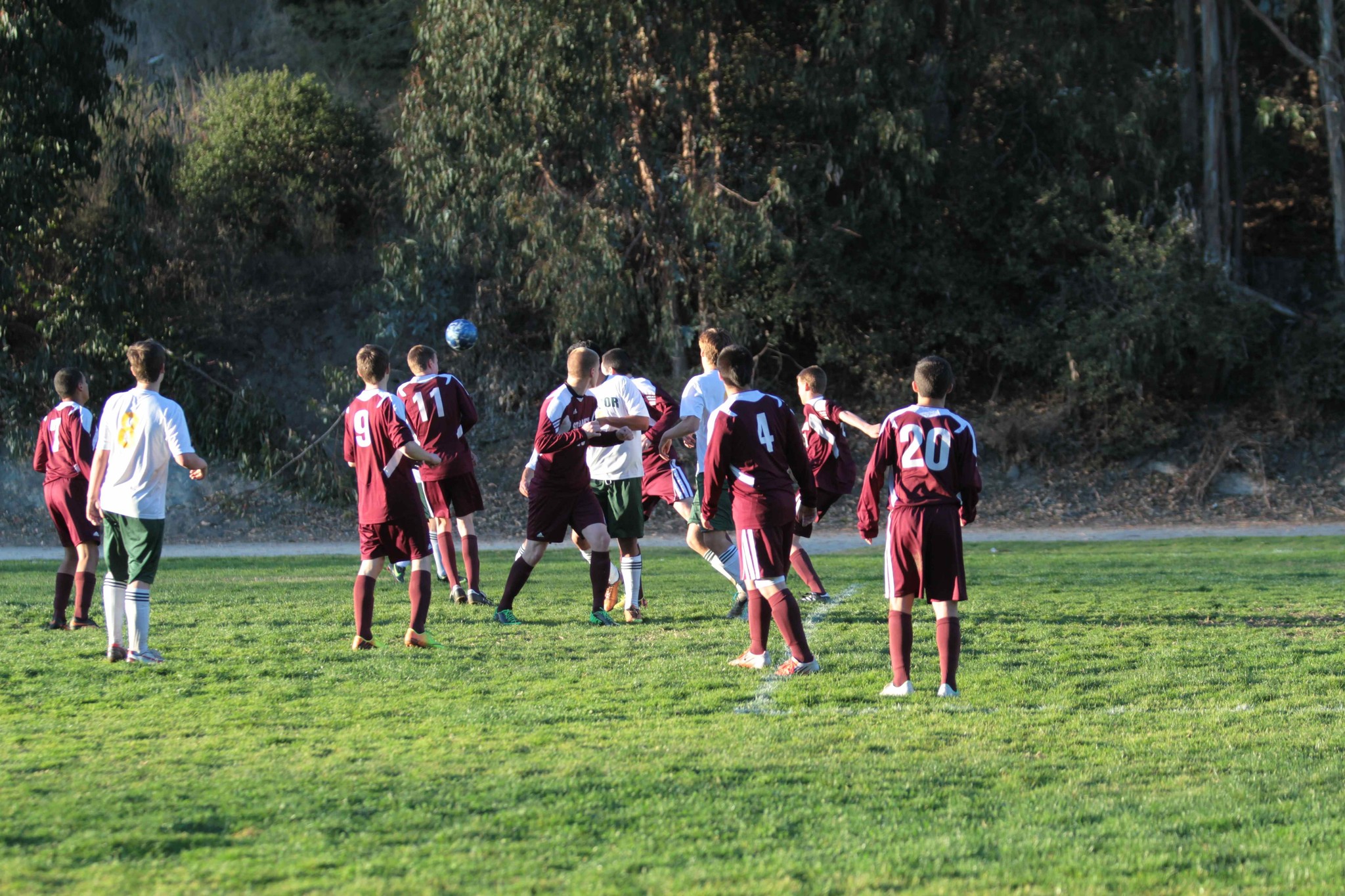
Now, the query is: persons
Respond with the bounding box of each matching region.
[490,340,748,627]
[698,344,822,678]
[344,343,468,649]
[33,368,103,631]
[85,338,207,666]
[784,365,883,602]
[855,355,982,696]
[397,345,494,607]
[657,327,749,618]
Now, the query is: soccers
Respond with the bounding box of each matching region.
[445,318,479,351]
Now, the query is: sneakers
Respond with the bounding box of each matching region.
[437,572,468,583]
[937,684,959,697]
[797,590,830,603]
[727,591,748,619]
[50,619,69,630]
[386,562,406,583]
[449,585,468,604]
[352,635,390,651]
[774,656,819,674]
[603,568,623,612]
[126,648,165,663]
[590,607,621,626]
[468,589,494,605]
[69,617,99,630]
[107,644,128,662]
[624,604,643,622]
[880,680,913,696]
[492,607,522,624]
[613,594,647,609]
[404,628,445,648]
[728,647,771,669]
